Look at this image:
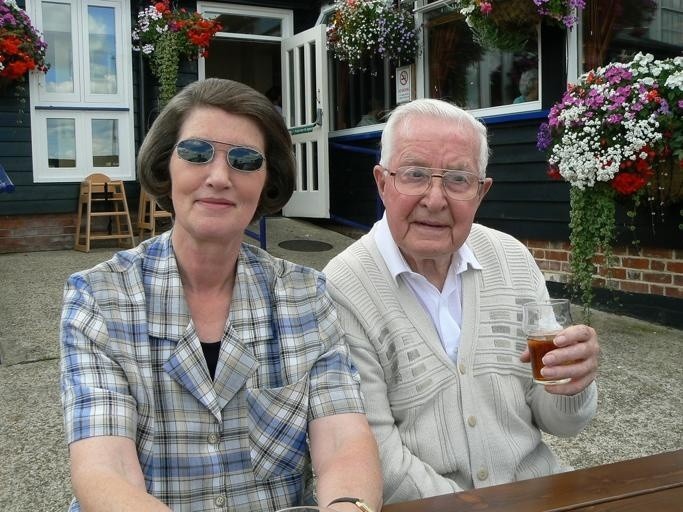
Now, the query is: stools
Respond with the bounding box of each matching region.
[135,182,174,241]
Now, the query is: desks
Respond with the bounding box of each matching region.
[373,450,683,511]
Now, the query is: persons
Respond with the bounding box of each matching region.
[317,97,601,504]
[57,78,386,512]
[262,81,281,116]
[354,96,387,127]
[511,66,541,105]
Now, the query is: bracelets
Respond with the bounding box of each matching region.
[326,496,373,512]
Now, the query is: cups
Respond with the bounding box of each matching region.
[521,298,577,388]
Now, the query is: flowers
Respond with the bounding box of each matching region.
[440,0,587,58]
[325,1,422,81]
[128,1,224,116]
[0,0,51,86]
[535,52,682,328]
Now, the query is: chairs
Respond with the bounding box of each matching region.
[73,173,135,251]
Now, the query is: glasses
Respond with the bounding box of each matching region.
[384,166,489,202]
[164,138,271,172]
